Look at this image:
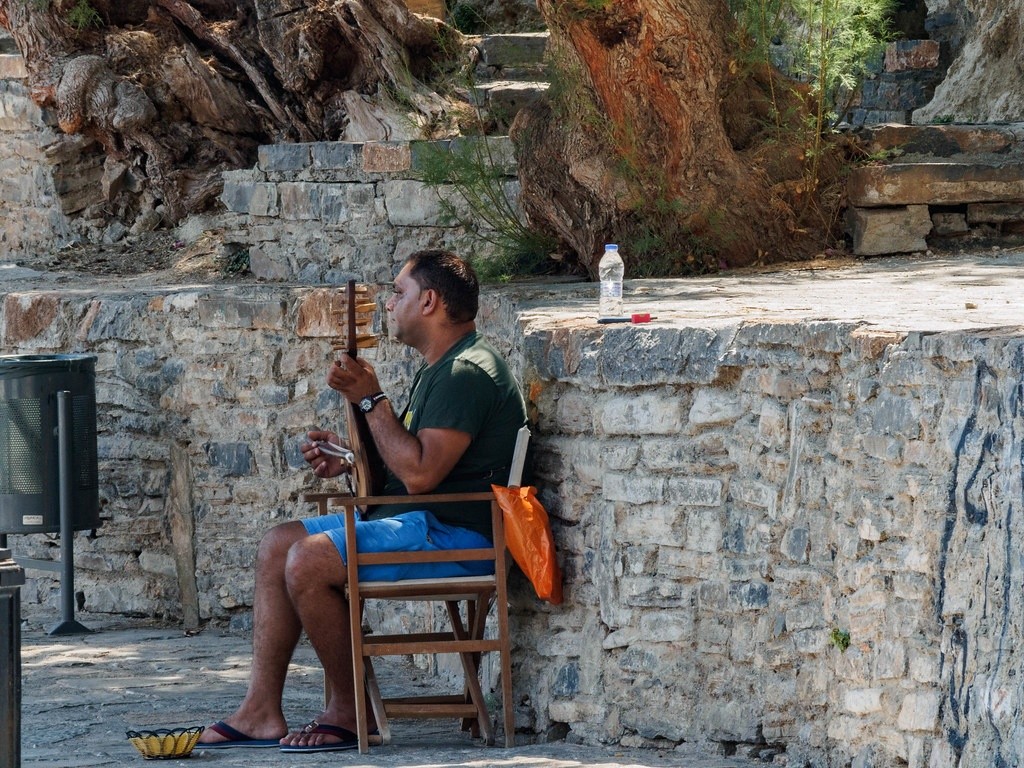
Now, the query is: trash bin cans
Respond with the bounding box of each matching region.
[0,353,104,535]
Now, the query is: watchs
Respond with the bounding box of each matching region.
[359,391,388,415]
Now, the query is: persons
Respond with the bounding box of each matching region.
[194,248,533,752]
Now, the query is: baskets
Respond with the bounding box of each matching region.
[125,726,205,759]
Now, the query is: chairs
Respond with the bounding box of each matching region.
[304,429,532,755]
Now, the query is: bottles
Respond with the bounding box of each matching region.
[599,244,625,318]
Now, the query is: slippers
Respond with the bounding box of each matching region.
[279,721,381,753]
[193,721,287,748]
